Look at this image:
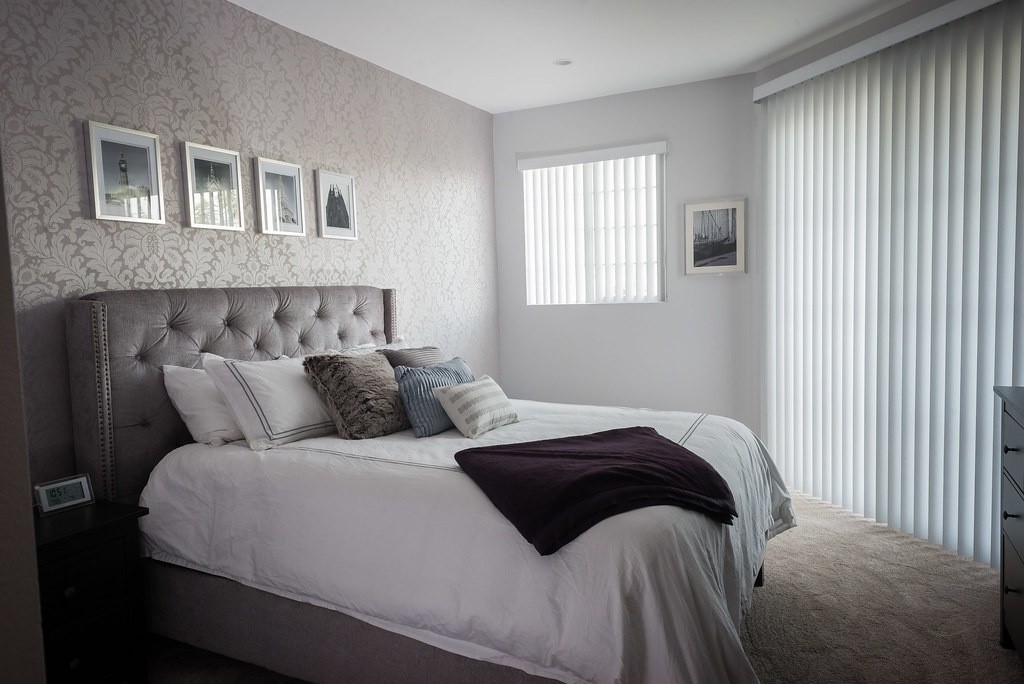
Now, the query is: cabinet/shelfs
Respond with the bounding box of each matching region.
[992,385,1024,653]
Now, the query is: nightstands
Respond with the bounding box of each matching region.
[32,497,149,684]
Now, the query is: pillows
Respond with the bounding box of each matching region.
[161,341,519,451]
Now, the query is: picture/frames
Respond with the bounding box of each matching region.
[253,156,307,237]
[683,196,750,275]
[179,142,245,233]
[83,119,166,225]
[315,169,358,241]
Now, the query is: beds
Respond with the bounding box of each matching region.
[63,284,796,684]
